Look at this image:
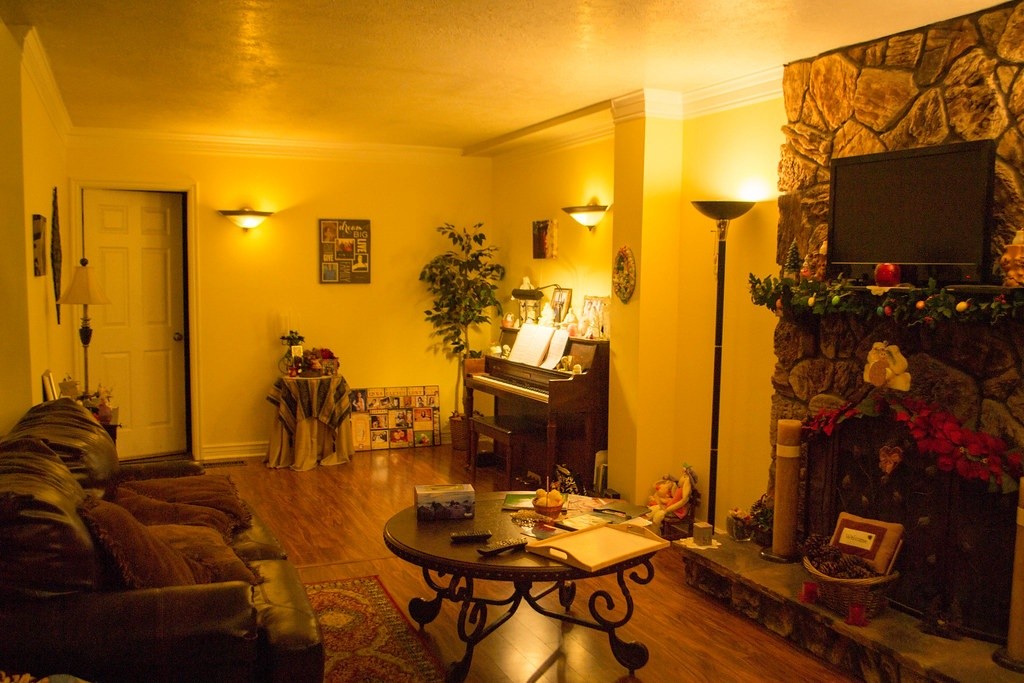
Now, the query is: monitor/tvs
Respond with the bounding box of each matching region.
[825,137,996,288]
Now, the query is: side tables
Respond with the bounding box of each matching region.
[94,406,119,447]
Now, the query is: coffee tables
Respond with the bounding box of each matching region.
[384,490,660,683]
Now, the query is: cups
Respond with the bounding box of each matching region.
[295,358,302,375]
[289,368,297,377]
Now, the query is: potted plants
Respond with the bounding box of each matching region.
[418,221,506,451]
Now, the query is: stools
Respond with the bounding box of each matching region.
[468,414,547,490]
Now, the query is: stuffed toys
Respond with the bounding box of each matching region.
[646,463,699,533]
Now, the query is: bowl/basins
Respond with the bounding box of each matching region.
[501,319,514,327]
[532,498,564,519]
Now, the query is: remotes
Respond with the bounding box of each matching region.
[477,539,528,556]
[450,530,492,542]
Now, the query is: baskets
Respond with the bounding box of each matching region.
[532,475,564,520]
[803,556,900,619]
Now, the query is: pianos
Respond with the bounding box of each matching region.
[463,326,610,497]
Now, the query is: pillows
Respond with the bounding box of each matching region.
[78,498,215,588]
[120,474,251,529]
[105,486,237,546]
[148,522,265,587]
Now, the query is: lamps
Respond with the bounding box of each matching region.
[689,199,758,526]
[54,266,112,401]
[562,205,609,231]
[220,210,273,231]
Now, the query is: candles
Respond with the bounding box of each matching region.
[773,420,802,555]
[1002,478,1023,664]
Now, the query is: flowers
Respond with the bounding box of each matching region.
[748,230,1023,491]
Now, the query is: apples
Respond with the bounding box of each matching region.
[874,262,901,288]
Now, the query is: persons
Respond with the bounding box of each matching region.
[353,393,435,444]
[561,308,577,331]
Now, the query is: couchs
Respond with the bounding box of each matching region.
[0,397,326,683]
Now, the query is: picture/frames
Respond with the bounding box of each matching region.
[42,370,59,401]
[319,219,370,284]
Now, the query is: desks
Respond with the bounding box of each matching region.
[266,375,352,471]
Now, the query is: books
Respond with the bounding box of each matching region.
[503,493,569,511]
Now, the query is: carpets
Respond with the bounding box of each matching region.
[301,576,449,683]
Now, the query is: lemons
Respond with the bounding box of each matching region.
[535,489,563,507]
